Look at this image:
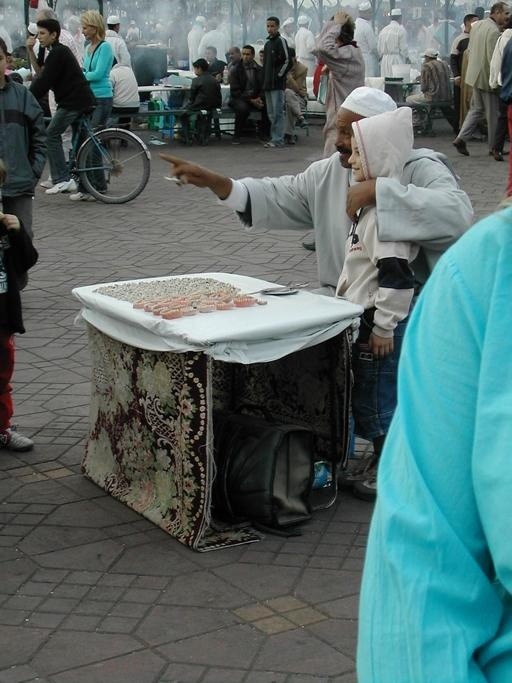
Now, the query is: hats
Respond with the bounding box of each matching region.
[298,16,309,24]
[391,9,402,16]
[196,16,205,22]
[280,17,294,28]
[424,48,438,58]
[358,1,371,11]
[28,23,38,34]
[341,87,398,118]
[330,15,355,37]
[107,16,120,24]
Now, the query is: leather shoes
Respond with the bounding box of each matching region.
[489,149,509,161]
[452,138,469,156]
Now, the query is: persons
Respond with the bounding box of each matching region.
[356,203,511,683]
[334,106,431,503]
[1,1,512,291]
[0,153,42,451]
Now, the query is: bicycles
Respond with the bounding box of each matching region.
[27,96,154,200]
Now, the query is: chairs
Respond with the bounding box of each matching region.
[285,78,309,136]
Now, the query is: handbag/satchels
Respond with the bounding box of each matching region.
[211,410,318,535]
[318,75,329,104]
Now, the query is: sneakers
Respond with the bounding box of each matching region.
[40,176,106,201]
[233,135,240,144]
[300,118,307,127]
[338,454,381,499]
[264,133,297,147]
[0,428,34,450]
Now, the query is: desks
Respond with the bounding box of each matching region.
[139,85,231,143]
[385,79,454,101]
[73,270,364,551]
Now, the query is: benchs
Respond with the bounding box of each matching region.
[120,109,189,148]
[395,101,452,136]
[289,111,326,126]
[190,107,269,146]
